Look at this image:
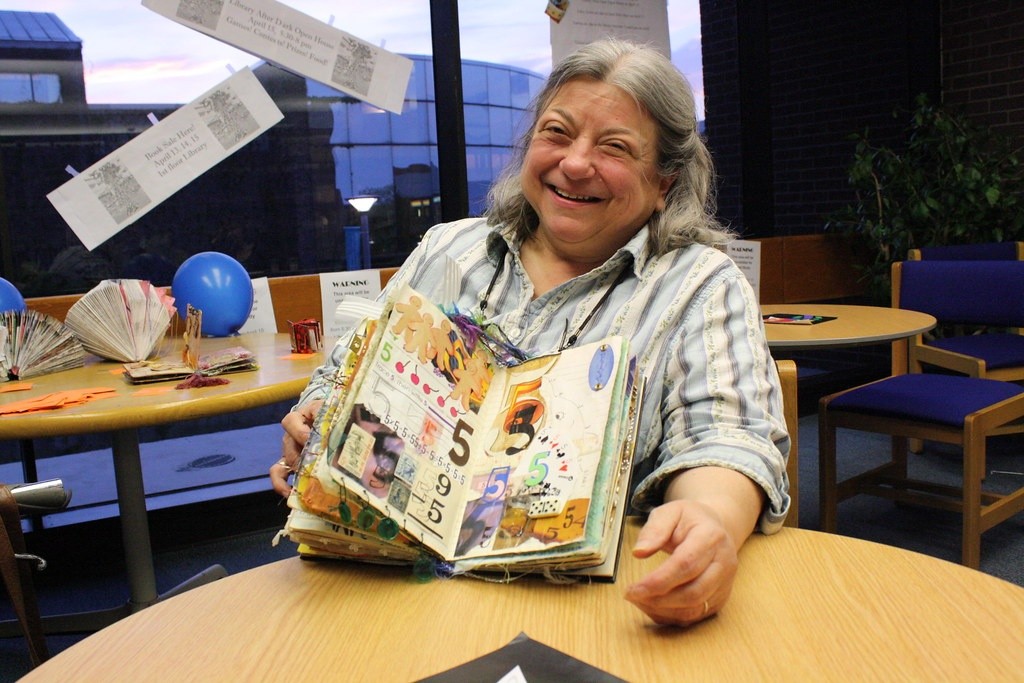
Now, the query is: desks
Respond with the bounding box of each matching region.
[751,298,938,355]
[14,507,1024,683]
[0,328,334,618]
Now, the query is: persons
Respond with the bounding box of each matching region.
[267,38,792,634]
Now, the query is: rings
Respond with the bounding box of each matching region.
[705,601,709,616]
[276,458,287,465]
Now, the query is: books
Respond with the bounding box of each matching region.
[275,282,646,586]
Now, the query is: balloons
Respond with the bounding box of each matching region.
[172,251,255,337]
[0,277,24,315]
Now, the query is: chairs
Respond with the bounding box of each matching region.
[815,258,1023,569]
[907,243,1024,455]
